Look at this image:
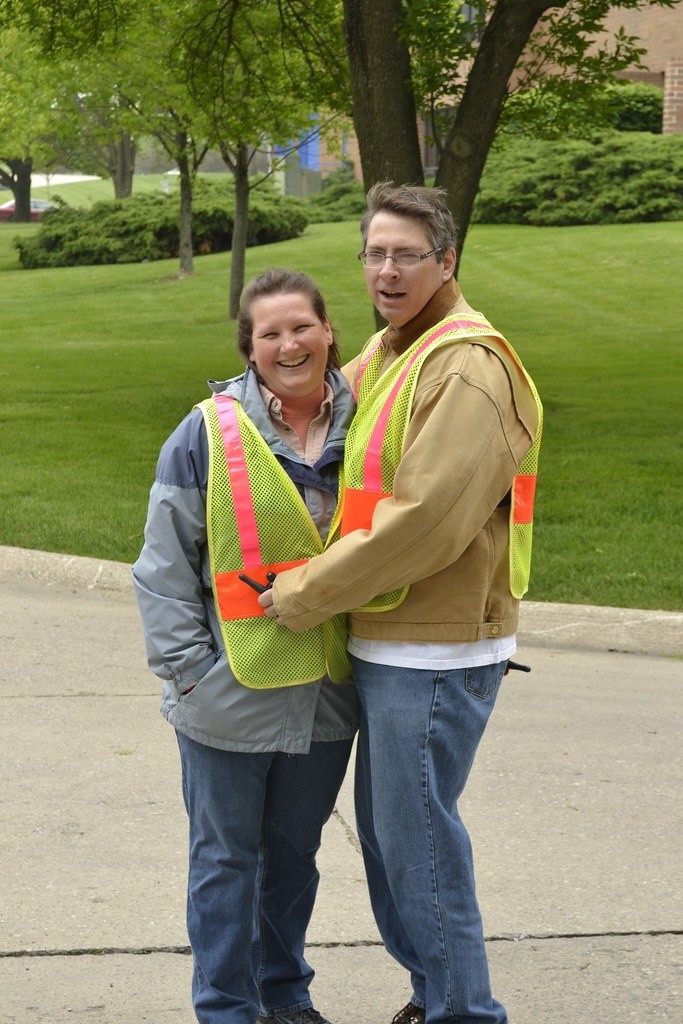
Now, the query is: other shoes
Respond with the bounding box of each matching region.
[392,1003,426,1023]
[257,1007,332,1024]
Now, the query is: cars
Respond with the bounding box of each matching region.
[166,168,180,175]
[0,199,56,222]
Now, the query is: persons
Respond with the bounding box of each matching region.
[257,180,544,1024]
[133,269,360,1024]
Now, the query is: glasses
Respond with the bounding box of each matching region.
[358,248,442,266]
